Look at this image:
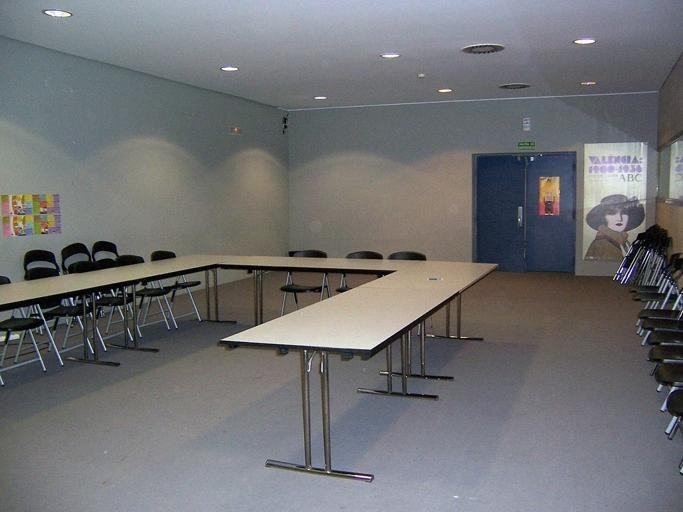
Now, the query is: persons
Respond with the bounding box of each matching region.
[540,179,556,215]
[585,194,645,262]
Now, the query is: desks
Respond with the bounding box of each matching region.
[0,254,121,386]
[219,286,439,483]
[76,254,237,354]
[220,254,423,342]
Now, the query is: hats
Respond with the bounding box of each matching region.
[585,195,644,231]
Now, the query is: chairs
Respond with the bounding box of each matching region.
[0,275,47,373]
[613,224,672,287]
[23,250,60,334]
[634,253,681,294]
[387,251,426,335]
[653,360,683,434]
[635,285,683,335]
[647,327,683,346]
[647,343,683,375]
[62,259,117,355]
[92,240,129,317]
[358,260,500,382]
[60,243,94,327]
[143,249,203,331]
[641,258,683,310]
[106,251,172,338]
[639,318,683,346]
[336,251,384,293]
[91,257,136,352]
[280,248,331,316]
[668,388,683,444]
[13,265,95,366]
